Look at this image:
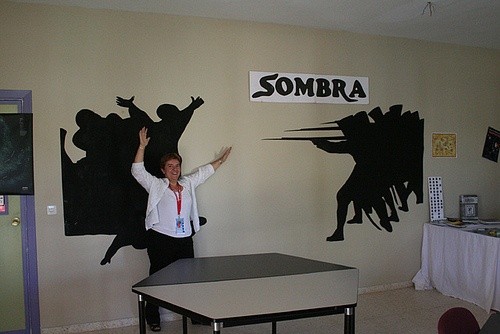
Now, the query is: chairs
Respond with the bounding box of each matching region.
[437,308,500,334]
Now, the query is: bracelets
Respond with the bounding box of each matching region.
[139,144,145,150]
[219,159,222,164]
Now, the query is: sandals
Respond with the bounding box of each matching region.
[150,323,161,332]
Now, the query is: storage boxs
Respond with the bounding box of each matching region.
[459,194,479,219]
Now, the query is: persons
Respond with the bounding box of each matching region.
[131,125,232,332]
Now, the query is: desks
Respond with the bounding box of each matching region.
[132,252,359,334]
[411,218,500,314]
